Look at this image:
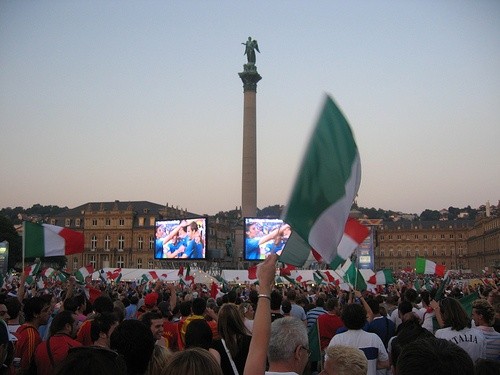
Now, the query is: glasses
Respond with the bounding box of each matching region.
[302,346,312,357]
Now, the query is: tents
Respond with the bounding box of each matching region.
[72,267,220,290]
[222,269,378,285]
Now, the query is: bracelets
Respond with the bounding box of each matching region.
[358,295,363,299]
[258,294,271,299]
[433,307,440,310]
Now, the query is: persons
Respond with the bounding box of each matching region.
[0,289,391,375]
[152,253,279,374]
[156,221,204,258]
[390,268,500,375]
[246,222,292,259]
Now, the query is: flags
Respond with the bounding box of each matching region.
[366,268,393,284]
[279,91,372,293]
[23,262,195,288]
[210,281,236,303]
[248,260,267,279]
[23,221,85,257]
[416,256,446,278]
[457,291,478,318]
